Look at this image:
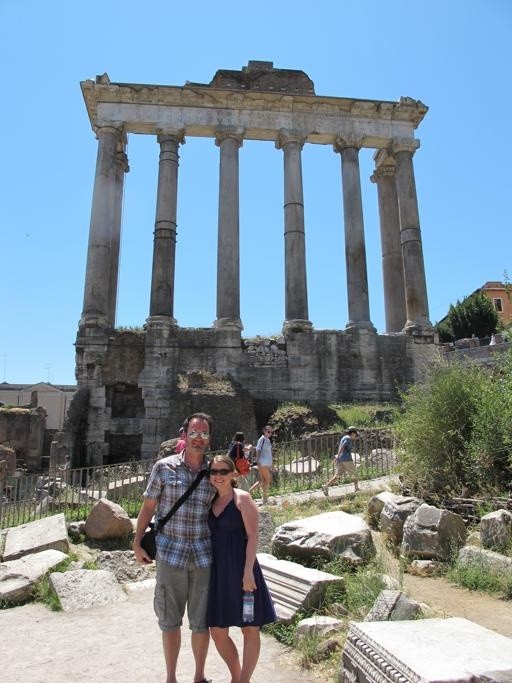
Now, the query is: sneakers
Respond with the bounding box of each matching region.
[322,484,329,496]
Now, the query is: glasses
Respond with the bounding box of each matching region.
[268,431,273,433]
[189,431,209,439]
[209,469,232,475]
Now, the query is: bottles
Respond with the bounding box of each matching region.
[242,591,255,623]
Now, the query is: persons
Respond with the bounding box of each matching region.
[321,428,361,496]
[249,425,277,505]
[59,455,69,481]
[131,411,219,682]
[208,454,277,682]
[176,426,186,454]
[226,431,252,490]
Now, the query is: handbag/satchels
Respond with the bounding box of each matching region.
[140,523,156,559]
[235,457,250,473]
